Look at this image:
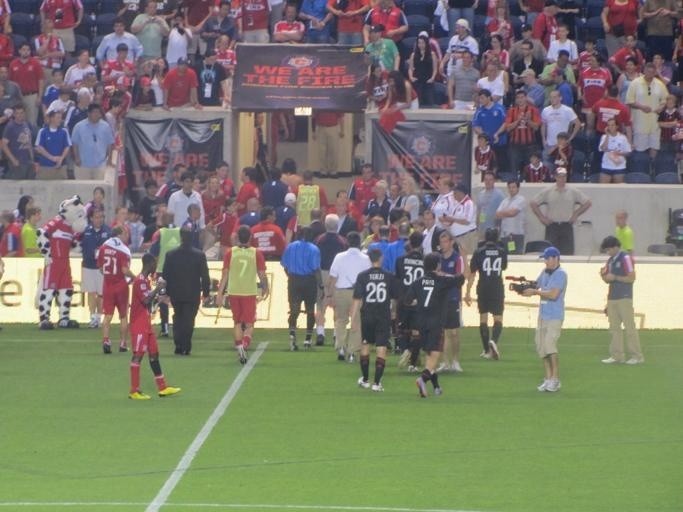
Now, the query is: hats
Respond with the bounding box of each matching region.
[450,183,469,195]
[368,23,384,32]
[539,246,561,259]
[177,56,189,66]
[519,69,535,78]
[554,167,568,177]
[284,192,296,204]
[455,17,472,33]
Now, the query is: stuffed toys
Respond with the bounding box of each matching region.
[35,195,88,329]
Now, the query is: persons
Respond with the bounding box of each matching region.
[77,204,111,328]
[388,175,419,226]
[208,198,241,261]
[96,225,136,353]
[114,178,207,258]
[291,169,329,236]
[346,163,379,213]
[168,170,206,229]
[274,192,297,245]
[494,178,527,255]
[281,226,325,352]
[531,166,592,257]
[368,225,391,264]
[10,195,34,225]
[0,210,24,259]
[83,187,105,221]
[382,222,412,276]
[123,206,146,253]
[201,175,226,226]
[475,172,507,243]
[328,231,372,363]
[313,214,349,345]
[420,210,443,256]
[429,179,459,232]
[598,235,645,365]
[475,0,683,185]
[435,230,466,374]
[161,227,210,356]
[280,158,302,190]
[404,239,471,398]
[214,226,270,365]
[611,210,635,257]
[0,0,144,180]
[129,254,182,399]
[260,167,288,206]
[216,165,238,201]
[248,204,290,263]
[131,1,482,111]
[253,111,270,175]
[351,247,402,393]
[20,207,47,257]
[236,166,261,216]
[465,226,508,361]
[231,197,263,247]
[517,247,567,392]
[438,182,477,255]
[301,187,437,255]
[364,179,391,219]
[112,205,132,248]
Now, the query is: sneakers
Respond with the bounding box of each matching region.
[435,363,449,371]
[357,377,370,388]
[433,387,442,396]
[625,357,645,365]
[545,379,560,392]
[128,390,152,400]
[87,318,101,329]
[304,337,312,345]
[537,379,551,392]
[371,382,385,391]
[103,340,112,354]
[479,350,492,359]
[601,356,625,364]
[415,377,429,397]
[158,387,181,398]
[119,341,127,353]
[408,365,419,374]
[316,334,325,345]
[347,352,354,362]
[488,340,501,360]
[337,347,345,361]
[451,360,463,372]
[160,327,171,338]
[397,348,412,369]
[288,334,297,352]
[239,346,247,365]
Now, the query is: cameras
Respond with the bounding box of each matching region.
[509,276,540,294]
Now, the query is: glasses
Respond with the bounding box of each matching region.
[647,86,652,96]
